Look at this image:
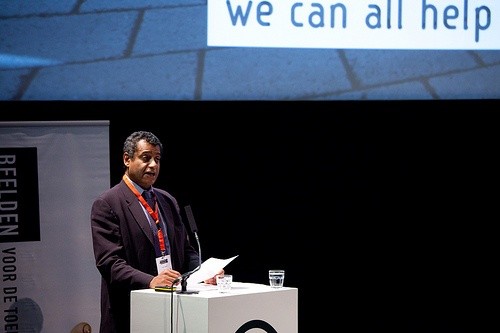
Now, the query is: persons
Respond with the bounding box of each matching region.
[90,130,202,333]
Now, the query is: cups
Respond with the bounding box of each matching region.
[216,274,232,293]
[269,270,285,288]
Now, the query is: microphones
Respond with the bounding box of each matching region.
[173,205,202,284]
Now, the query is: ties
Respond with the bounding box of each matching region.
[142,191,169,256]
